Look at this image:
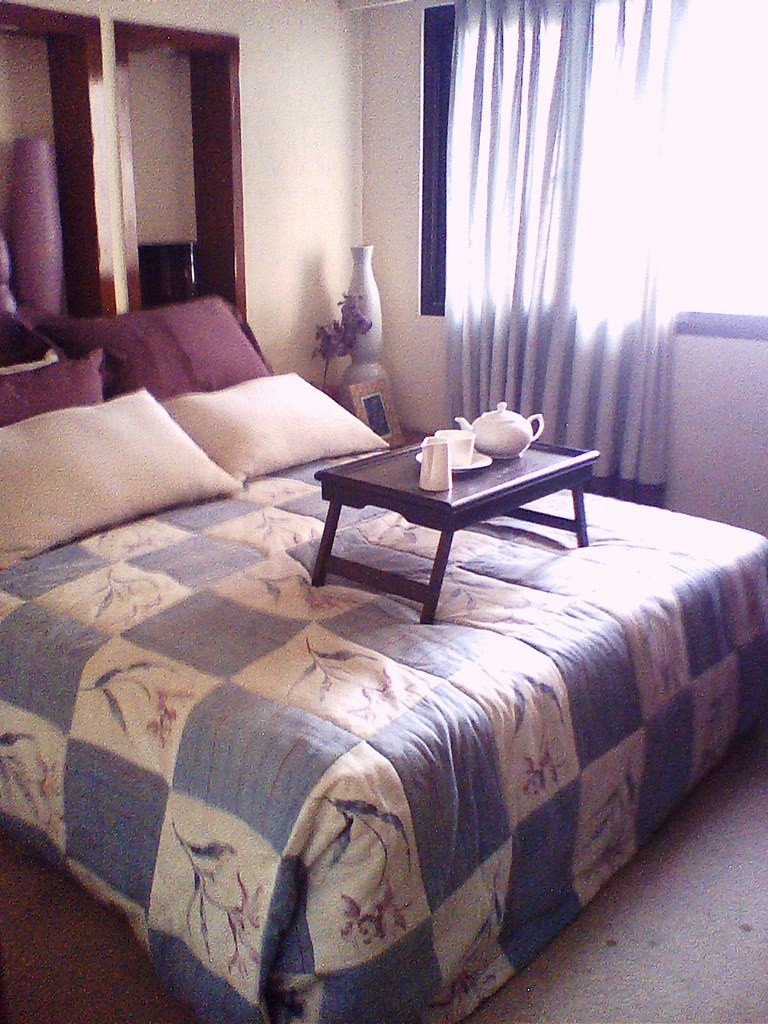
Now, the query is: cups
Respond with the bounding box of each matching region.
[419,437,452,491]
[435,430,476,466]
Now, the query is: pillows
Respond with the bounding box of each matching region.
[30,293,275,402]
[0,345,104,427]
[0,386,244,571]
[161,372,391,483]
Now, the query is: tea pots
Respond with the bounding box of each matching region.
[454,402,544,460]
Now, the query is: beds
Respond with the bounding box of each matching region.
[0,292,768,1024]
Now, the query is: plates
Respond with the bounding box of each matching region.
[416,452,492,473]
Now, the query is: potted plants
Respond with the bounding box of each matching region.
[311,293,373,402]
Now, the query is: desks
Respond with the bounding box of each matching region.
[310,442,601,625]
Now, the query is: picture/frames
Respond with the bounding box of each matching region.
[348,377,406,449]
